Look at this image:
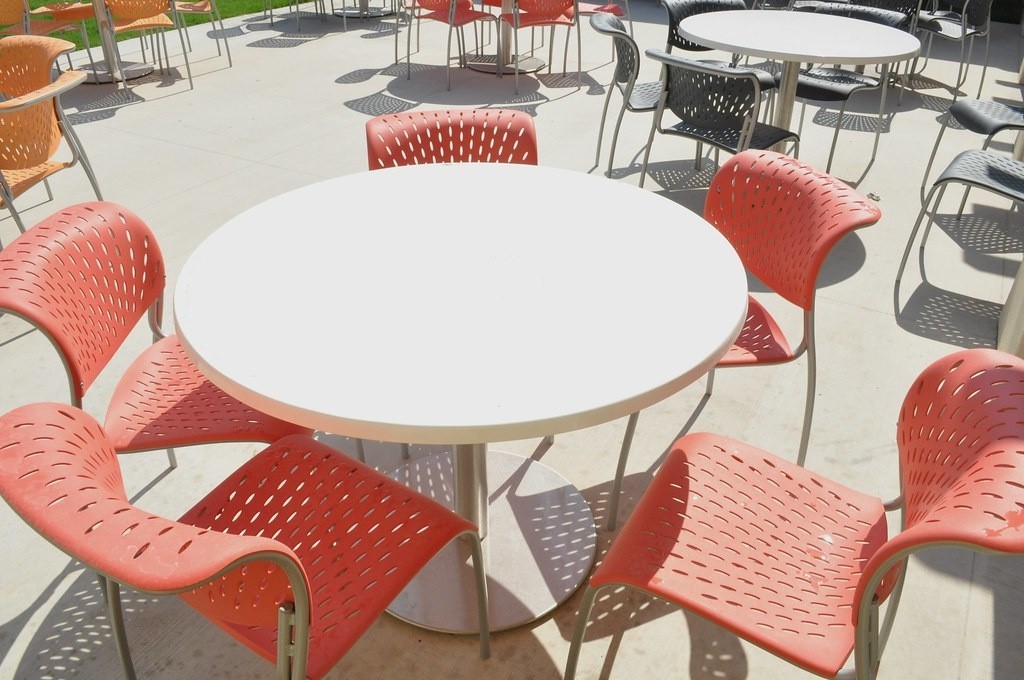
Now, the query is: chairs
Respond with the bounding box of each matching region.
[0,200,367,608]
[921,99,1024,220]
[0,70,104,249]
[774,2,907,177]
[589,12,734,180]
[0,0,100,86]
[395,0,625,95]
[101,0,193,100]
[366,107,555,458]
[544,149,883,533]
[0,402,491,680]
[564,347,1024,680]
[31,0,109,63]
[152,0,232,75]
[895,149,1024,282]
[909,0,993,103]
[639,49,800,188]
[662,0,774,170]
[0,34,76,199]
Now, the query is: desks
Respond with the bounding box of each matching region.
[676,9,922,156]
[172,163,747,635]
